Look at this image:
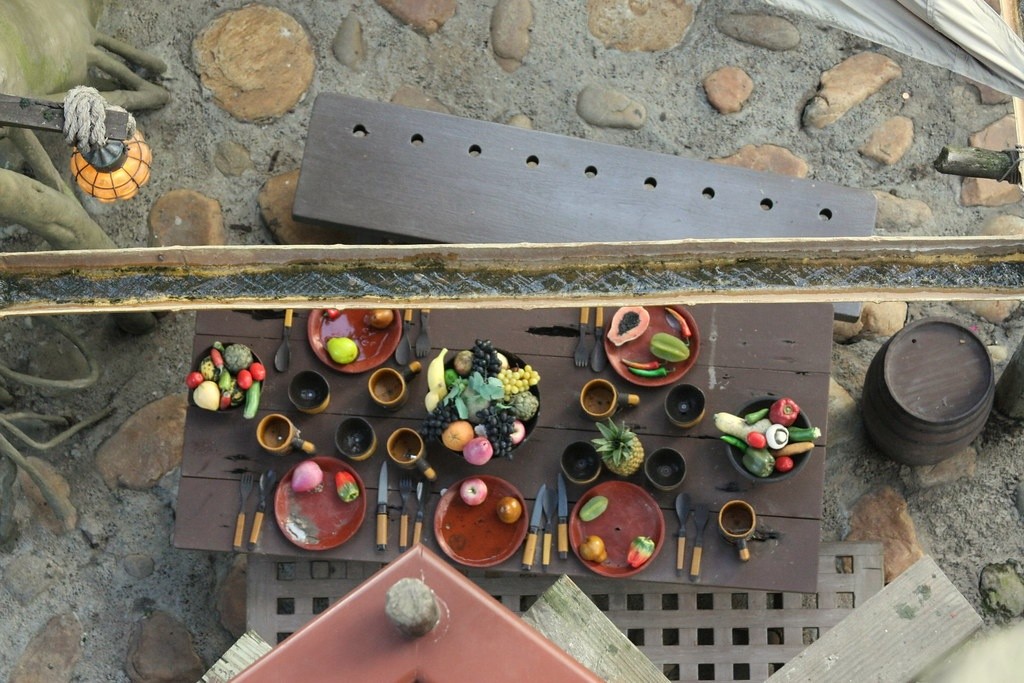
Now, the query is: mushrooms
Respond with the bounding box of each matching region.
[766,424,789,449]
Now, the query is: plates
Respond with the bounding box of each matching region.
[307,309,402,374]
[434,474,529,567]
[603,305,700,386]
[569,481,665,578]
[274,456,366,550]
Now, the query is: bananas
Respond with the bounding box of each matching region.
[427,348,449,400]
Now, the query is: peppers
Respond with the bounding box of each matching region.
[335,471,359,503]
[211,342,231,410]
[719,408,821,478]
[627,535,655,568]
[324,310,342,319]
[621,358,675,378]
[665,307,692,345]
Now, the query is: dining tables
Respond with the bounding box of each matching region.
[174,302,835,598]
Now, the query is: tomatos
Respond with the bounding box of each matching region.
[186,363,265,389]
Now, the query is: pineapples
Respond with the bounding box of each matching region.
[589,417,644,477]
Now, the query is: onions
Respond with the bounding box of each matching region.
[292,461,323,492]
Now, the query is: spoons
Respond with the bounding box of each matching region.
[676,492,691,576]
[589,307,607,372]
[542,487,558,567]
[275,308,293,372]
[249,469,277,550]
[412,479,431,545]
[395,309,413,365]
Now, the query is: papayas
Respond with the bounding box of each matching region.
[607,306,650,346]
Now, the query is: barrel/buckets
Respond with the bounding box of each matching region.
[862,316,995,467]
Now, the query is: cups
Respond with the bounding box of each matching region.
[664,384,707,428]
[387,427,438,482]
[288,370,330,414]
[644,447,687,491]
[256,414,316,456]
[334,416,378,461]
[559,440,602,486]
[719,500,757,561]
[580,379,640,421]
[368,361,422,411]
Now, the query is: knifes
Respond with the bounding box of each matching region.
[521,484,546,570]
[557,474,568,559]
[377,461,387,550]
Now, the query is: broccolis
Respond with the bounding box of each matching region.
[224,343,253,373]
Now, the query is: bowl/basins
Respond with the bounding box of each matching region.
[190,342,264,412]
[428,348,541,459]
[726,392,813,482]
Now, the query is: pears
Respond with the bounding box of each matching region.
[579,536,608,563]
[326,337,358,365]
[368,309,394,329]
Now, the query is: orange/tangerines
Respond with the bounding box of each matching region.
[496,497,522,523]
[440,420,474,452]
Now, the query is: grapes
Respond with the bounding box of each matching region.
[418,338,540,461]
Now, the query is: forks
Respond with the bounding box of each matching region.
[689,504,710,580]
[416,309,431,357]
[575,307,589,367]
[399,472,412,553]
[233,472,253,549]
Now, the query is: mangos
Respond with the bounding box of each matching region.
[579,495,608,522]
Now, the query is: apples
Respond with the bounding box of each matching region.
[460,477,487,506]
[512,420,526,445]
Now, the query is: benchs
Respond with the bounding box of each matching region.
[292,89,878,323]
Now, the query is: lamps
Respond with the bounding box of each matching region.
[70,130,153,204]
